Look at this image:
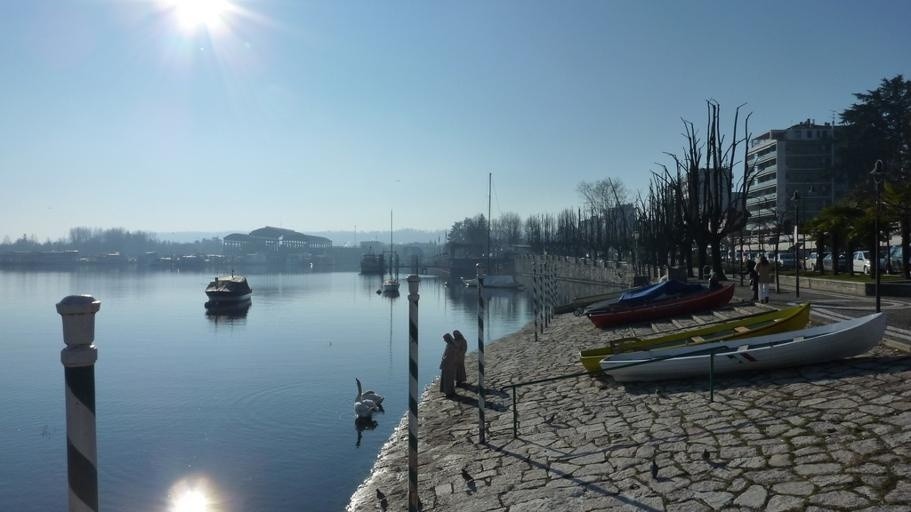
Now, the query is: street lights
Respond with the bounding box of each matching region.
[869,158,886,314]
[788,188,803,302]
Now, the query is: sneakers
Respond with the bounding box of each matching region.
[750,297,769,304]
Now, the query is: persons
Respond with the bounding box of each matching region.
[439,333,459,398]
[753,254,772,304]
[454,330,467,387]
[741,260,759,301]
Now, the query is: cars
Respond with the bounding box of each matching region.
[719,243,906,279]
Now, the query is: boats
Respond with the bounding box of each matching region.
[204,297,251,322]
[203,268,252,303]
[382,278,400,292]
[383,289,399,298]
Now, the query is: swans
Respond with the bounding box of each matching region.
[352,376,384,418]
